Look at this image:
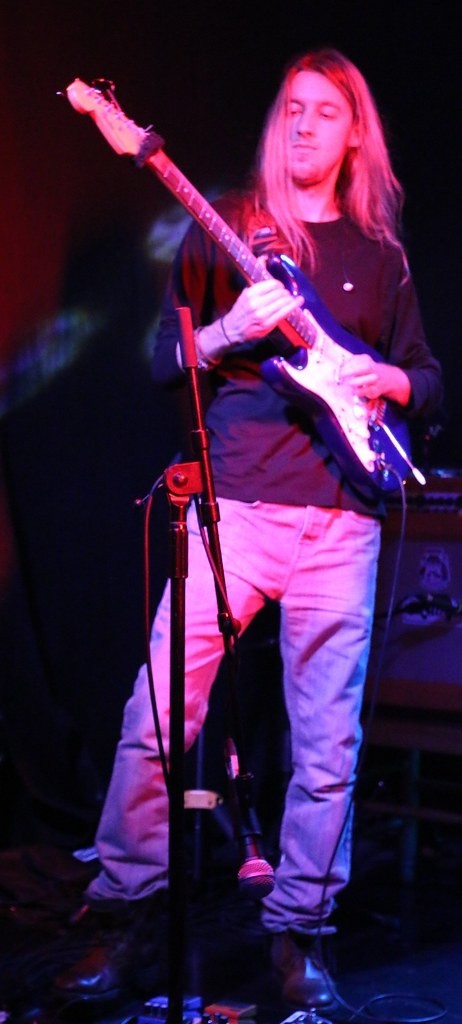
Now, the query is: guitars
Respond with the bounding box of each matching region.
[55,77,427,505]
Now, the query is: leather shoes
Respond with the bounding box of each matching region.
[56,905,156,1002]
[255,937,335,1012]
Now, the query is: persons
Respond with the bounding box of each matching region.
[48,46,444,1016]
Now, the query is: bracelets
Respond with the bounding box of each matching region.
[193,326,222,366]
[194,348,214,372]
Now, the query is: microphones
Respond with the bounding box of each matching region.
[174,303,207,429]
[220,738,277,900]
